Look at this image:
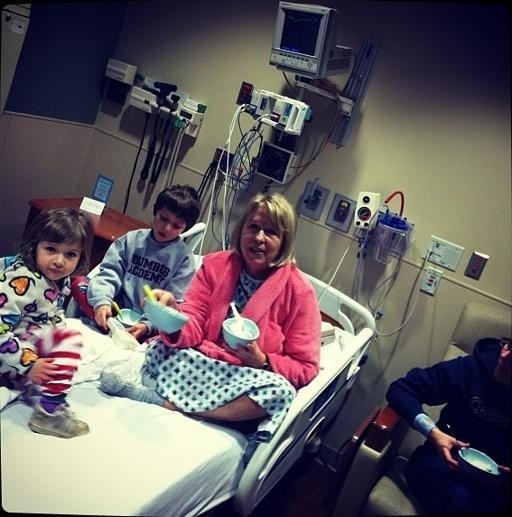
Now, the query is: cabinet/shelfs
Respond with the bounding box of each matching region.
[26,190,147,272]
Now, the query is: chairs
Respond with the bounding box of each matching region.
[342,295,509,515]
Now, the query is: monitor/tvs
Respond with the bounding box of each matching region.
[269,1,352,79]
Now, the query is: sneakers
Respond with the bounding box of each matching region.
[27,408,90,438]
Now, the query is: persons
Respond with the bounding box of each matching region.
[95,190,325,426]
[70,183,196,344]
[384,334,512,517]
[0,203,89,439]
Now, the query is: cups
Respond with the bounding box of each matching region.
[372,209,410,265]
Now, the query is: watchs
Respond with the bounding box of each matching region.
[260,353,270,370]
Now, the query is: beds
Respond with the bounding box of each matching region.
[0,220,377,515]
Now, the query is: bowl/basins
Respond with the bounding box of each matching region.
[459,446,502,476]
[120,308,141,326]
[223,317,261,348]
[144,296,192,336]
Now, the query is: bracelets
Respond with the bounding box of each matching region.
[411,413,437,439]
